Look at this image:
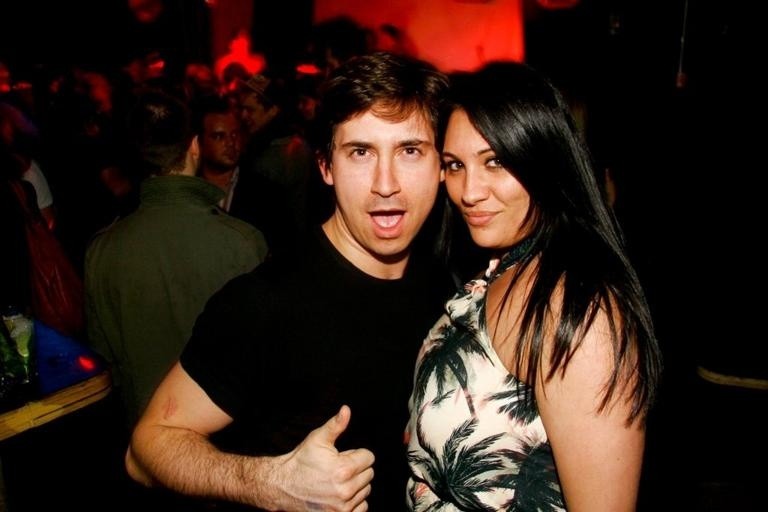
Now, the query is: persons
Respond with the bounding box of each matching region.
[401,61,646,512]
[126,51,465,512]
[327,16,373,71]
[192,101,281,231]
[83,91,267,411]
[1,135,58,319]
[226,74,314,189]
